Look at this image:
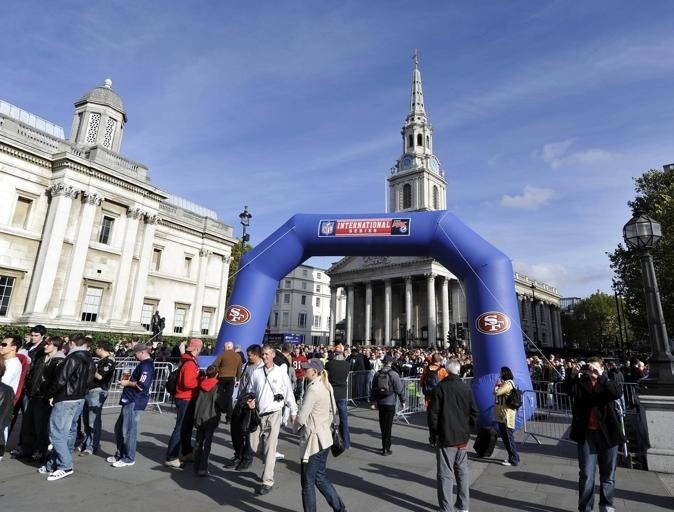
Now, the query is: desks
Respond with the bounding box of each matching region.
[530,283,541,348]
[239,206,252,256]
[622,208,673,395]
[611,279,631,358]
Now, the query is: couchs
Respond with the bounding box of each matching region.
[447,335,450,343]
[457,323,462,338]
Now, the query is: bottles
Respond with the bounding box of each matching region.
[607,362,616,366]
[432,354,443,363]
[335,343,344,355]
[300,358,324,372]
[129,343,148,357]
[186,339,203,351]
[380,354,395,363]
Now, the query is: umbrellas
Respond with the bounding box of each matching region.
[256,483,272,490]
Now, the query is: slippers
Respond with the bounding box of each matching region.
[376,369,394,399]
[422,365,442,397]
[504,381,523,410]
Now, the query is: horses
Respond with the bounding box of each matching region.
[580,364,590,371]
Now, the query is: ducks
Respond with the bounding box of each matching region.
[430,441,437,448]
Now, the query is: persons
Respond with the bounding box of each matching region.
[322,343,352,448]
[165,338,204,468]
[192,365,224,477]
[419,352,451,448]
[157,317,165,340]
[492,366,520,466]
[291,357,346,512]
[562,357,627,511]
[113,336,173,362]
[428,358,479,512]
[36,333,92,480]
[212,341,241,424]
[106,343,156,467]
[369,355,406,455]
[151,311,162,341]
[294,341,652,416]
[224,341,291,472]
[2,326,116,460]
[245,343,298,496]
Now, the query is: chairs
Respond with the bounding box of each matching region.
[47,469,74,481]
[197,469,210,476]
[383,449,392,456]
[80,448,96,457]
[453,505,469,512]
[153,452,288,468]
[32,450,42,460]
[504,459,519,464]
[74,445,85,452]
[501,462,514,466]
[11,446,24,455]
[37,465,54,474]
[107,455,121,462]
[112,459,136,468]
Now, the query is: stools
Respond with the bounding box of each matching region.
[258,412,276,417]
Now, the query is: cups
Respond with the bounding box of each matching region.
[0,343,12,347]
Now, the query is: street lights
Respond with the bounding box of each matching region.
[165,359,197,396]
[330,425,345,457]
